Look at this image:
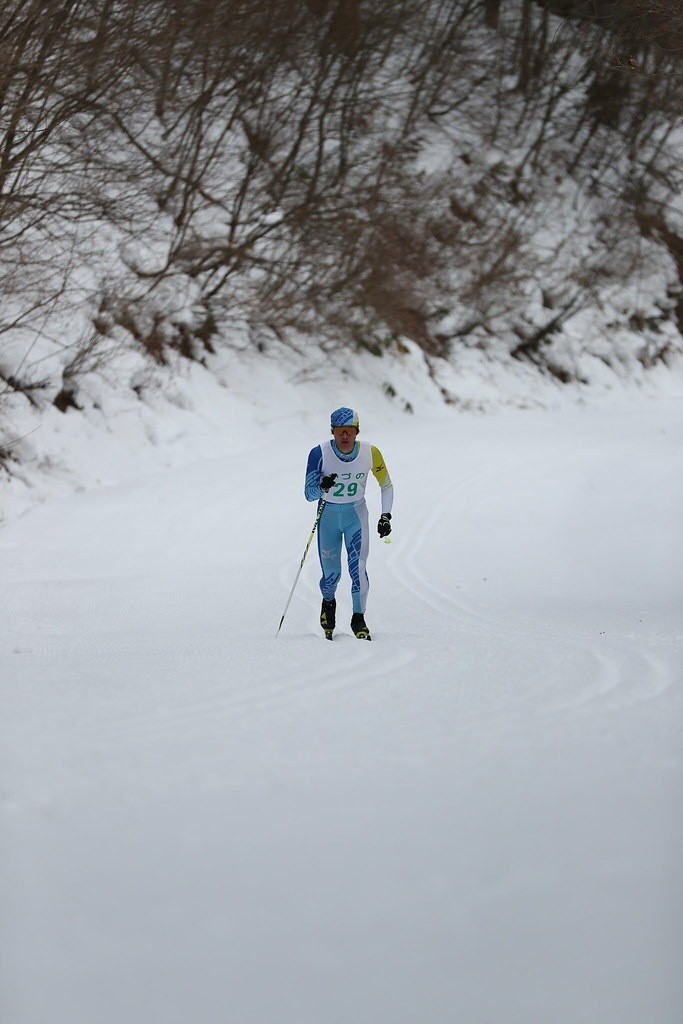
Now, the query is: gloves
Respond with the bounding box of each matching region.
[320,472,338,490]
[378,513,392,538]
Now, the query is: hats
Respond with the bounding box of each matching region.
[331,407,359,428]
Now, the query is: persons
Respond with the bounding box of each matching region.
[306,407,393,640]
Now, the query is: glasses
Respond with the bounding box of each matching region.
[332,426,359,436]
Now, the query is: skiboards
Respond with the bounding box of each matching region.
[322,627,372,642]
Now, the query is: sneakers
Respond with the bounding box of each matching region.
[351,613,369,637]
[320,598,336,629]
